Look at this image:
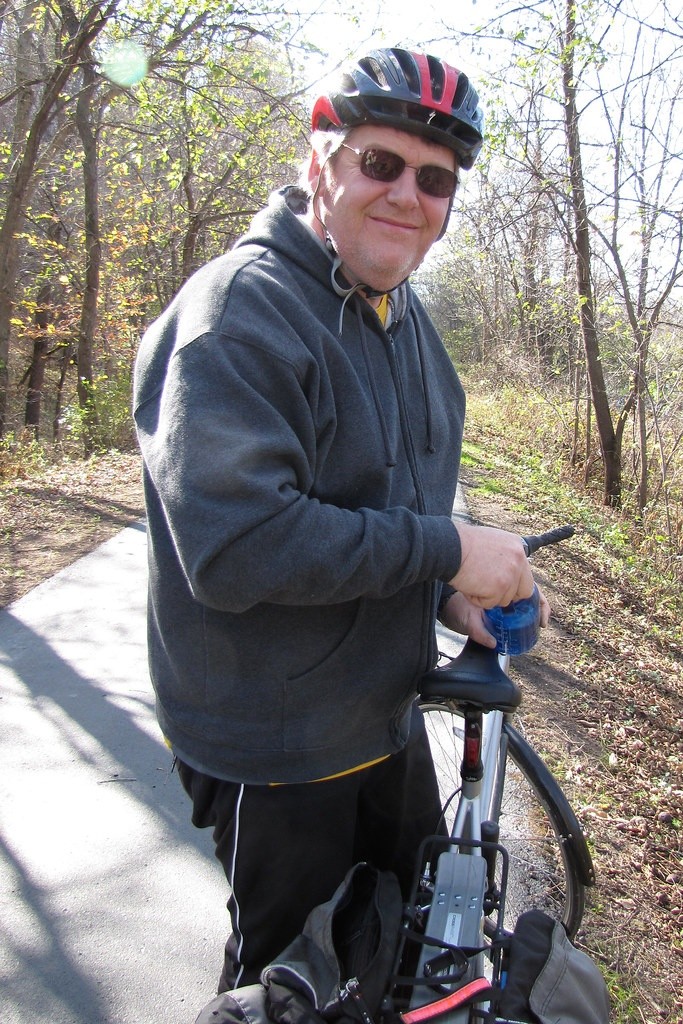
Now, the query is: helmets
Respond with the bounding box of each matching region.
[312,47,484,170]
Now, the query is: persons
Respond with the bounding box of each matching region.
[124,46,552,994]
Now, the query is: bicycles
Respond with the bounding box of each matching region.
[367,527,594,1023]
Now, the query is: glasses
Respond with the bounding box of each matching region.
[339,143,460,198]
